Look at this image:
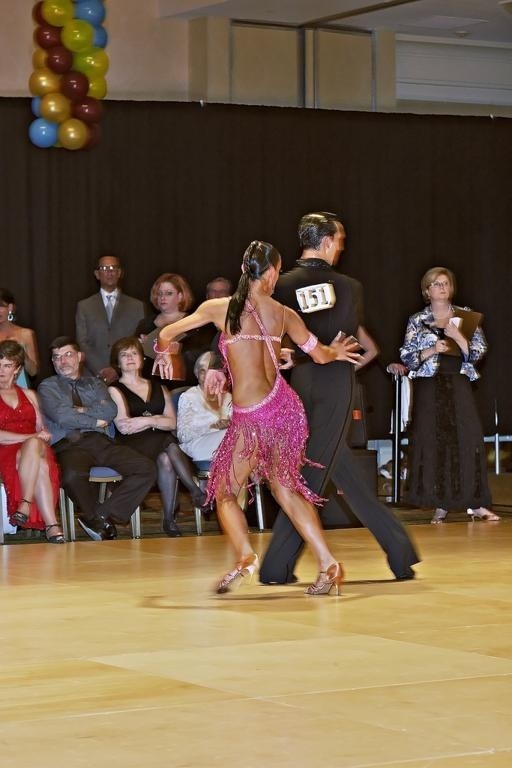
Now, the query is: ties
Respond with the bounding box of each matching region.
[70,381,82,406]
[105,295,113,325]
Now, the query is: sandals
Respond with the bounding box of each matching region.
[47,525,64,543]
[12,499,30,522]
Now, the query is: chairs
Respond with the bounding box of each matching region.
[1,385,264,541]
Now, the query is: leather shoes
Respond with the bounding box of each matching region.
[163,520,181,537]
[77,510,116,540]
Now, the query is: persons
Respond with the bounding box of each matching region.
[399,265,503,524]
[152,241,365,599]
[1,254,290,543]
[258,210,425,586]
[357,354,408,439]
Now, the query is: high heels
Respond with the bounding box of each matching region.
[217,554,257,593]
[431,509,449,524]
[467,508,500,520]
[306,563,342,595]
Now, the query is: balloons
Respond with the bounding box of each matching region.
[28,0,111,154]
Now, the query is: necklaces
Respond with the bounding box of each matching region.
[429,302,451,319]
